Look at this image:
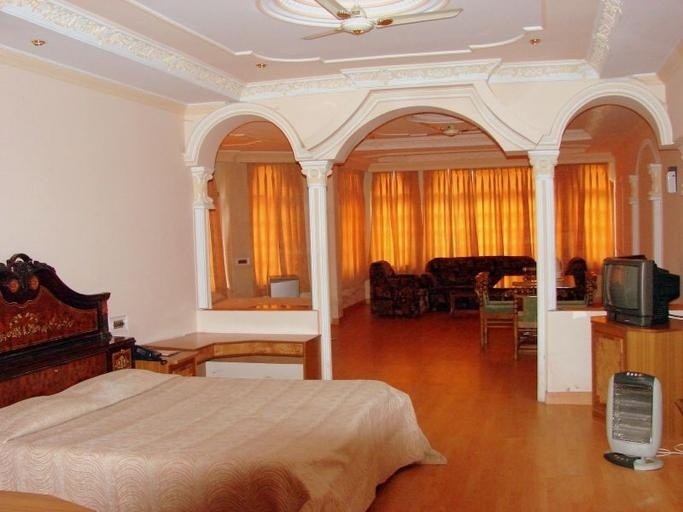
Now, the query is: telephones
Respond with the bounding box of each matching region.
[132,345,161,360]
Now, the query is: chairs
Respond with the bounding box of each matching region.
[368,259,429,318]
[472,256,598,363]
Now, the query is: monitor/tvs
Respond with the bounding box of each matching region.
[603,257,680,327]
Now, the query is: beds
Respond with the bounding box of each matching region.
[0,253,425,512]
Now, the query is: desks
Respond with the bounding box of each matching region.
[148,332,322,380]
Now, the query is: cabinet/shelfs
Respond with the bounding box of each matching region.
[587,312,682,439]
[133,349,200,377]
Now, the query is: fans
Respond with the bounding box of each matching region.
[417,118,482,138]
[298,0,464,43]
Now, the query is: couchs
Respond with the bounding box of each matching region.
[420,256,536,314]
